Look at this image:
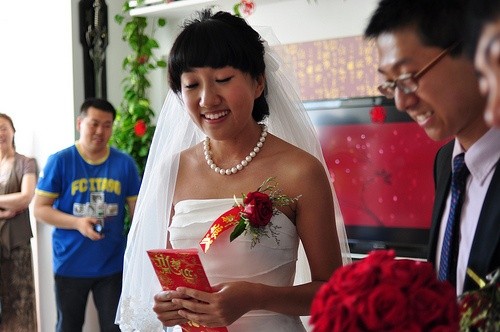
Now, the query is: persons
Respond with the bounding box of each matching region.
[117,7,351,332]
[0,113,39,332]
[34,98,142,332]
[364,1,500,331]
[474,0,500,136]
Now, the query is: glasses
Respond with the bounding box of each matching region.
[378,34,467,99]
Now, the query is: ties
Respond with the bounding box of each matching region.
[438,154,470,280]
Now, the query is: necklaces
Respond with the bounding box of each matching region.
[203,125,268,174]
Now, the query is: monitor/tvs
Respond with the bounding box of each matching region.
[297,96,453,262]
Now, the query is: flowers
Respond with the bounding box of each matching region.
[460,279,500,332]
[307,249,461,332]
[227,175,304,250]
